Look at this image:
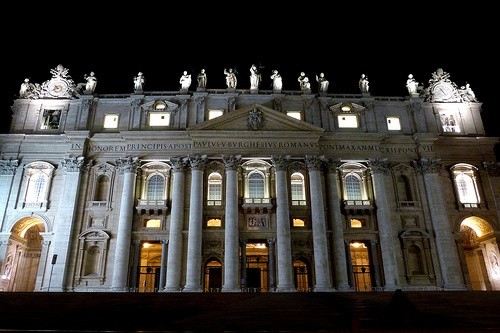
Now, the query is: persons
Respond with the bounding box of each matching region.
[297,72,310,90]
[197,69,207,88]
[405,74,419,93]
[179,71,191,89]
[224,68,238,89]
[359,74,370,92]
[270,70,283,90]
[249,64,262,89]
[316,73,329,92]
[133,72,145,90]
[84,71,97,93]
